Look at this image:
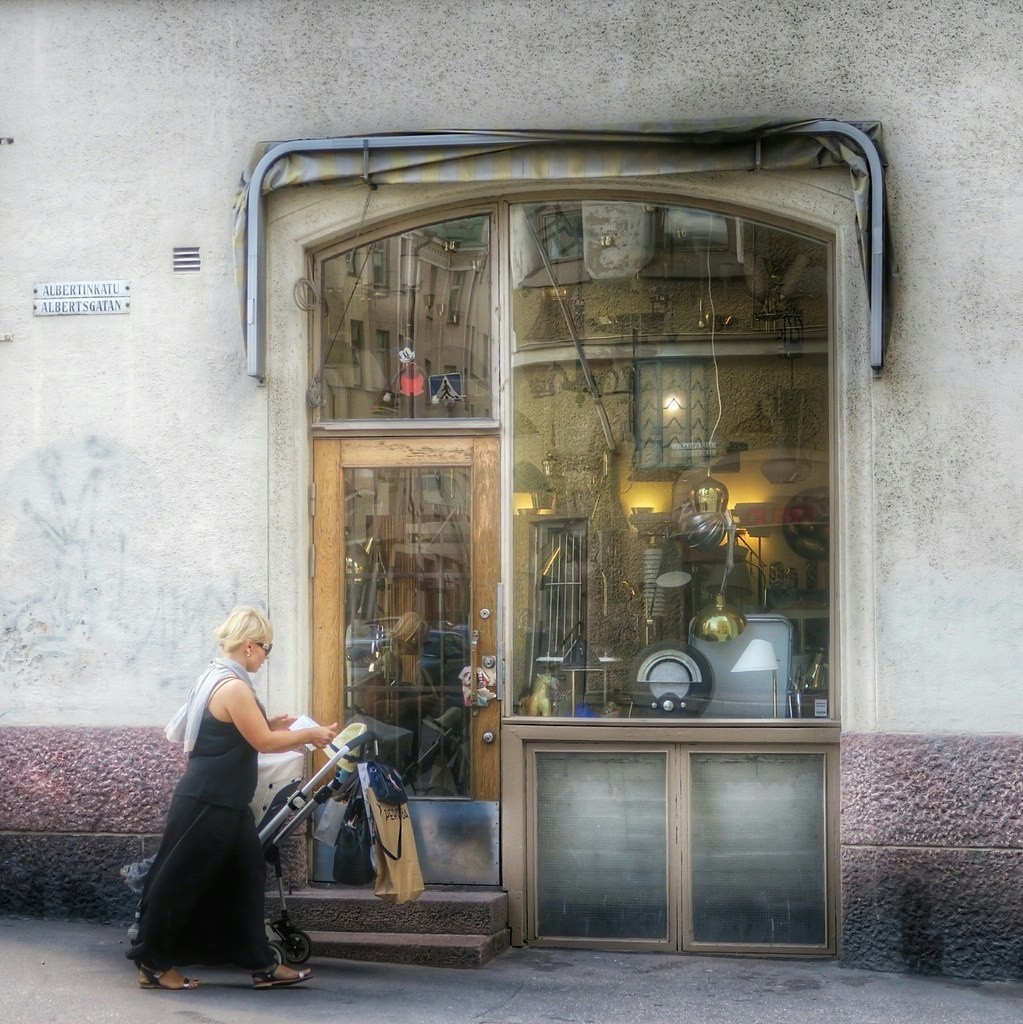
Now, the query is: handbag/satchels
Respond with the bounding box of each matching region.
[367,759,408,806]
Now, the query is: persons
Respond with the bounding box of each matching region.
[364,612,442,752]
[132,606,339,991]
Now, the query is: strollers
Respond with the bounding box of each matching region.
[400,728,460,796]
[127,732,374,969]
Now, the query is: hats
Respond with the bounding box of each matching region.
[421,706,463,743]
[323,722,368,772]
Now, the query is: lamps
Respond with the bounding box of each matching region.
[727,639,780,719]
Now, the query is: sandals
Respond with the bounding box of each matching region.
[138,967,197,990]
[252,962,314,989]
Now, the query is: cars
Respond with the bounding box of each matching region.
[346,631,470,720]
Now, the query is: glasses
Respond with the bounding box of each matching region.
[407,638,429,649]
[255,641,273,656]
